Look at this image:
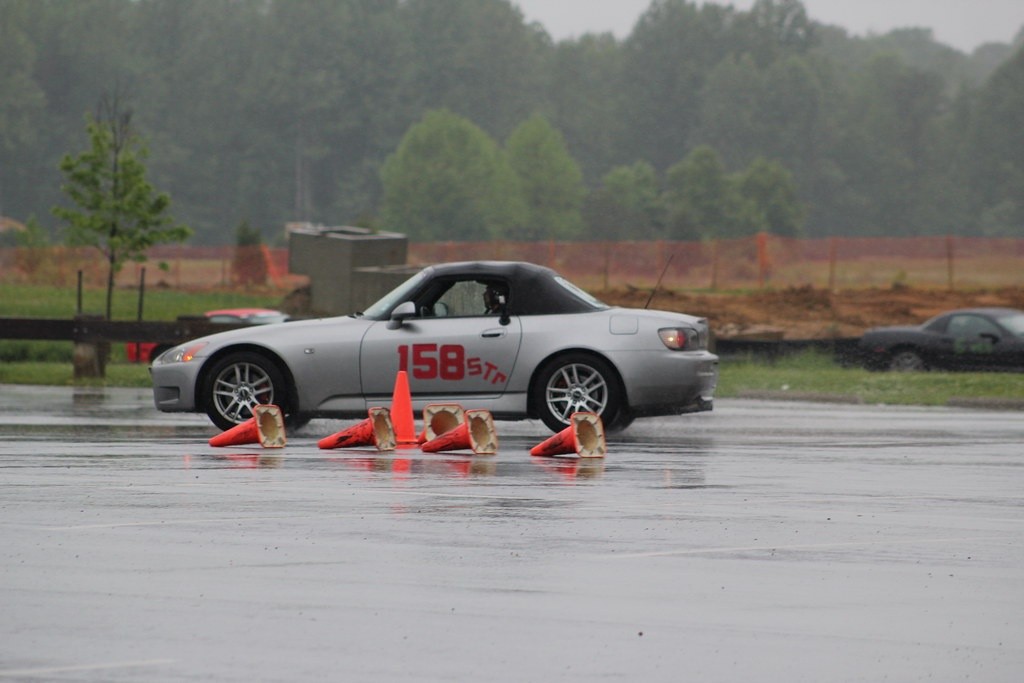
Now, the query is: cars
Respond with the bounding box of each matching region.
[859,307,1023,373]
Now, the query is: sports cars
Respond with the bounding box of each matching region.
[148,260,720,434]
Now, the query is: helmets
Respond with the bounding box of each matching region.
[487,281,509,304]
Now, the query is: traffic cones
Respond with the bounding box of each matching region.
[422,408,498,454]
[416,402,463,445]
[318,406,397,451]
[207,404,287,450]
[529,411,608,459]
[386,369,422,445]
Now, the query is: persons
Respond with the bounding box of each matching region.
[482,282,510,316]
